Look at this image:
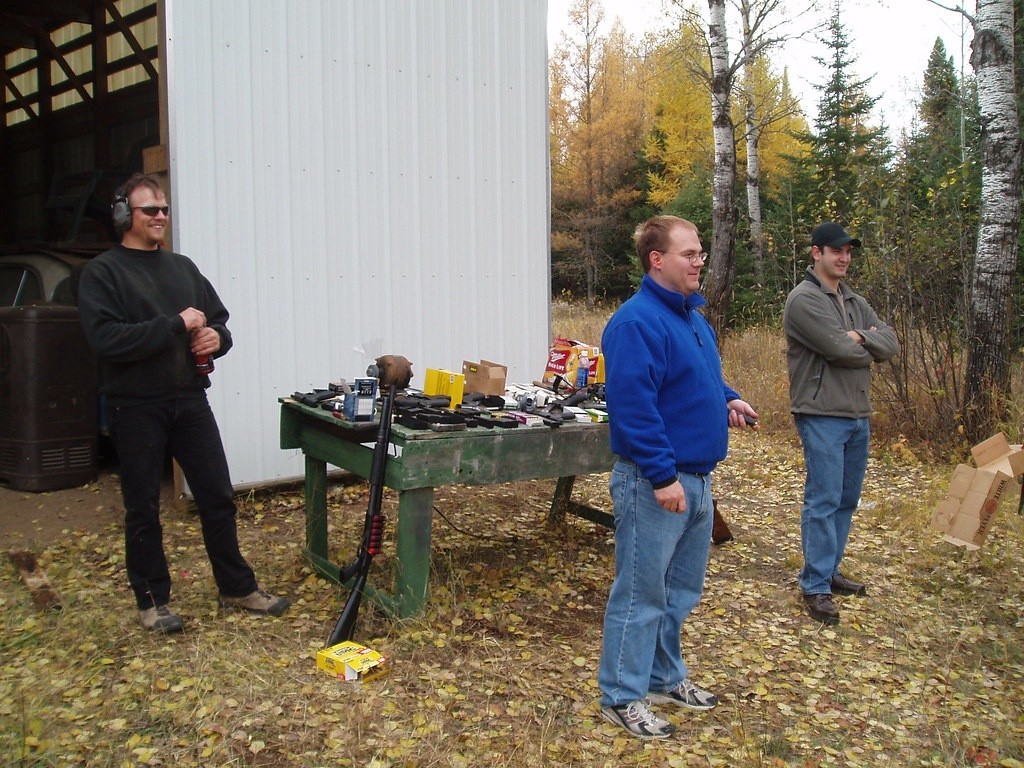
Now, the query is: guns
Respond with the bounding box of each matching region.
[711,497,734,546]
[291,379,607,431]
[324,377,398,649]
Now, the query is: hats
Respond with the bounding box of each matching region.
[812,221,862,249]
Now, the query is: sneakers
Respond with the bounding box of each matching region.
[648,680,717,711]
[139,603,180,633]
[600,696,676,741]
[218,588,289,618]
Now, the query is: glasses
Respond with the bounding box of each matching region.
[131,205,170,216]
[659,251,707,263]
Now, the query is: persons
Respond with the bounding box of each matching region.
[598,214,760,739]
[783,222,898,626]
[76,174,290,631]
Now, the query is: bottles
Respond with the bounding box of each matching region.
[575,351,589,389]
[192,325,215,374]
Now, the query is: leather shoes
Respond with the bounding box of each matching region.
[803,593,840,624]
[831,574,866,596]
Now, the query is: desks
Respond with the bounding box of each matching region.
[279,392,733,625]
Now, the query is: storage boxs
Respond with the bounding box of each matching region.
[317,640,388,684]
[425,368,465,410]
[462,359,507,397]
